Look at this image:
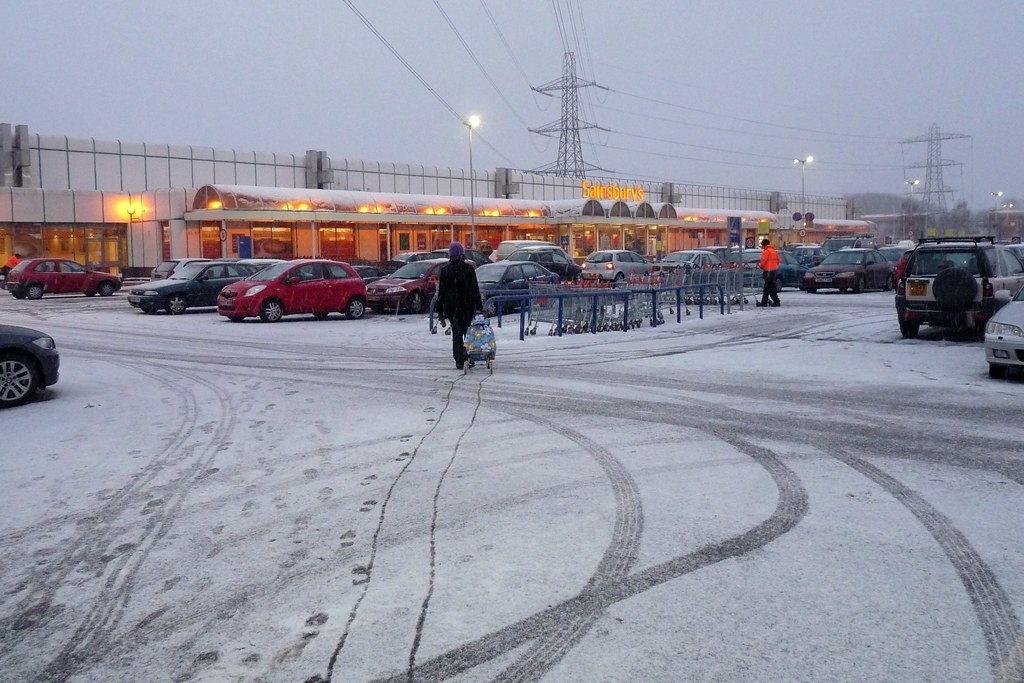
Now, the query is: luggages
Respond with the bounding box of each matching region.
[464,318,497,375]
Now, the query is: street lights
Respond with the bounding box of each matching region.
[126,207,135,276]
[991,192,1013,245]
[794,156,813,243]
[905,179,920,240]
[469,115,480,249]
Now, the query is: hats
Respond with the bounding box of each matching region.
[761,239,770,246]
[449,242,465,256]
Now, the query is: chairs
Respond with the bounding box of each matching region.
[45,265,49,272]
[220,271,225,278]
[839,255,849,263]
[930,260,954,277]
[206,270,214,279]
[289,269,302,282]
[968,257,978,276]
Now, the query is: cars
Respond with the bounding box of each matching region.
[985,281,1024,379]
[0,324,60,408]
[803,248,895,294]
[127,262,260,315]
[6,258,121,299]
[150,258,214,281]
[351,240,821,317]
[217,259,368,322]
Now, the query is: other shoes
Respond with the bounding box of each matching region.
[456,361,475,370]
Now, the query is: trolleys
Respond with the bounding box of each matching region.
[428,275,452,335]
[524,263,763,336]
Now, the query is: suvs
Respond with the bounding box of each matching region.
[813,232,884,267]
[895,236,1024,342]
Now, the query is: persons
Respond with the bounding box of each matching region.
[756,238,781,307]
[0,254,20,289]
[438,241,484,369]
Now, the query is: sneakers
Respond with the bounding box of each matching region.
[770,302,780,307]
[756,302,767,306]
